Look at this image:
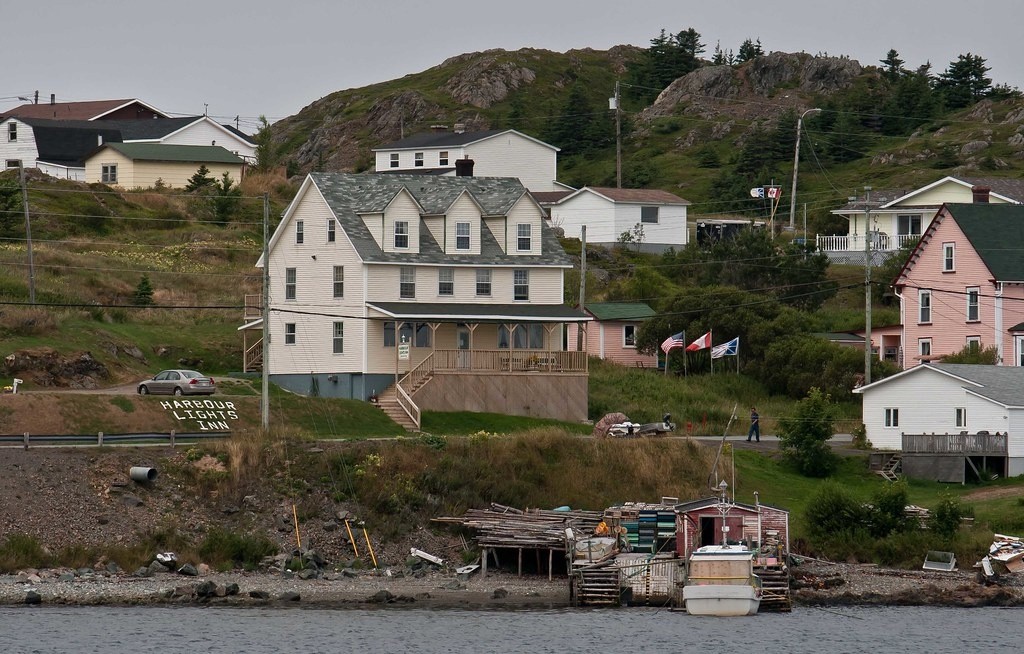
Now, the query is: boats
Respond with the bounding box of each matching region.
[575,537,618,560]
[609,422,676,438]
[684,480,760,617]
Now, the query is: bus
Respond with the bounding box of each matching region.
[697,219,781,252]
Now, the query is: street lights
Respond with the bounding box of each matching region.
[790,108,822,227]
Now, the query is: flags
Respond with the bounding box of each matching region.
[711,337,739,358]
[685,332,710,352]
[660,332,684,354]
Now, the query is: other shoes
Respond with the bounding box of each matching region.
[746,439,751,441]
[756,440,759,442]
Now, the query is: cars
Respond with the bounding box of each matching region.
[137,370,216,396]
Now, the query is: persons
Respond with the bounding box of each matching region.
[746,407,760,443]
